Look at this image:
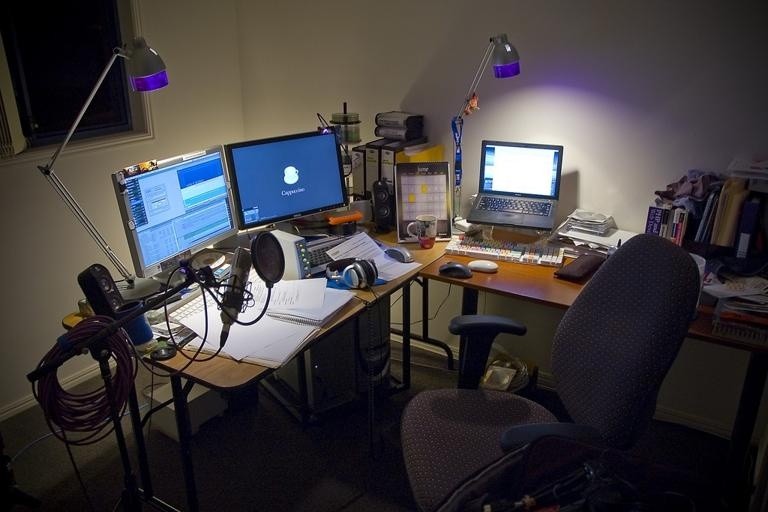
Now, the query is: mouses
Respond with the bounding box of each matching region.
[440,262,472,279]
[384,244,414,263]
[467,259,499,273]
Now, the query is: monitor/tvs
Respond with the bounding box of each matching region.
[224,126,348,231]
[111,144,239,288]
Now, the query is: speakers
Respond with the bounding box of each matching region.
[372,179,393,230]
[77,263,123,316]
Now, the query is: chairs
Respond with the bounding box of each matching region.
[403,232,700,512]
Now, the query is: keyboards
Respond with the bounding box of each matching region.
[444,235,566,268]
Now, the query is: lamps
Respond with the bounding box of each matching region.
[451,34,521,235]
[36,37,169,301]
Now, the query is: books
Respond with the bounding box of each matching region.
[374,126,424,142]
[552,158,767,348]
[351,144,367,203]
[396,142,444,167]
[393,161,453,243]
[365,137,392,212]
[152,274,357,370]
[374,110,424,129]
[380,138,427,217]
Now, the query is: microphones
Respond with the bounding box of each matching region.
[221,249,253,325]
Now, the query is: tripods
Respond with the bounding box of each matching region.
[26,261,216,512]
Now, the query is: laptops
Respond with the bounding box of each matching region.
[465,140,563,231]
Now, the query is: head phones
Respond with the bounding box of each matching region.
[326,257,379,289]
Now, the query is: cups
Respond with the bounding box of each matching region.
[114,301,153,345]
[407,214,437,250]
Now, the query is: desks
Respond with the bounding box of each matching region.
[418,227,768,458]
[62,231,454,512]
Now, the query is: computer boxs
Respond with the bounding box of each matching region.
[259,296,392,414]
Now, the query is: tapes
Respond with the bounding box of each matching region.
[328,221,356,235]
